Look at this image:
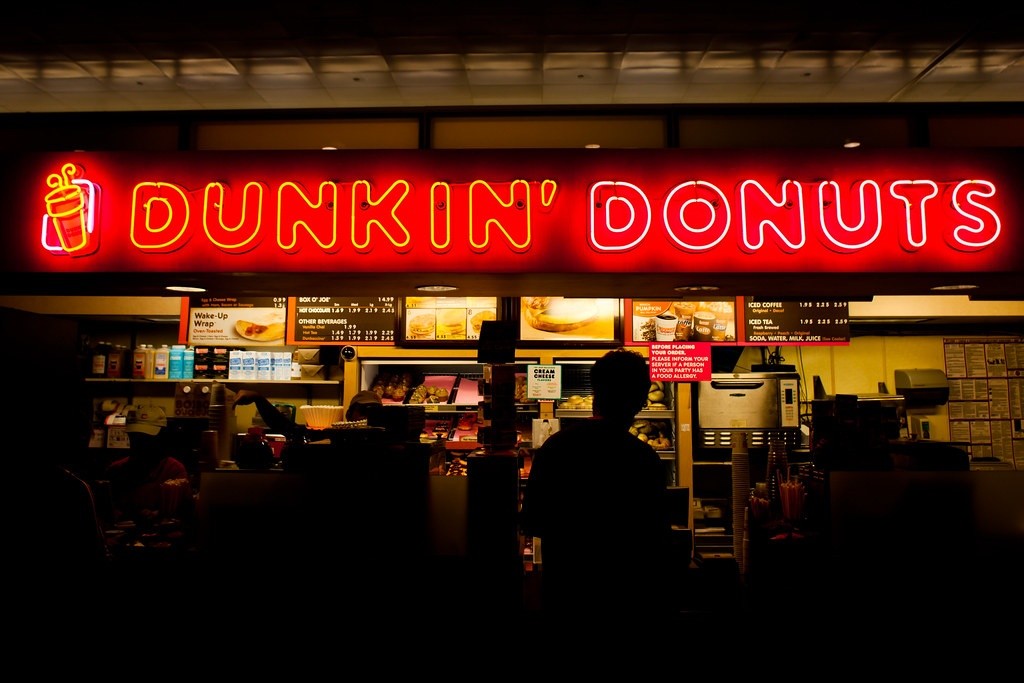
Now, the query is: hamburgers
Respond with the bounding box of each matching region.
[471,311,496,332]
[439,312,465,334]
[410,314,434,334]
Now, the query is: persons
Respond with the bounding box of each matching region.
[105,405,188,514]
[522,352,668,683]
[234,391,381,443]
[0,362,117,683]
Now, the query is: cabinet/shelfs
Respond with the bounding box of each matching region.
[342,346,695,563]
[84,376,341,475]
[693,461,735,564]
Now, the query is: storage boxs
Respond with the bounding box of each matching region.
[228,351,292,381]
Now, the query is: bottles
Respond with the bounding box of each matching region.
[754,480,770,502]
[523,540,533,576]
[767,440,790,496]
[899,418,909,438]
[92,341,194,379]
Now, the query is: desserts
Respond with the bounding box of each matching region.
[373,375,448,404]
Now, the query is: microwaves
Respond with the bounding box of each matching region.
[696,372,802,450]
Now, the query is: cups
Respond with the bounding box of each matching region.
[202,384,230,472]
[694,315,716,342]
[712,319,727,341]
[675,305,693,341]
[731,431,750,574]
[778,481,806,521]
[656,315,678,341]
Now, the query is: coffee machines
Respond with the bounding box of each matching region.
[896,370,970,445]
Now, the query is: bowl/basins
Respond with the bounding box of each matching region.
[237,433,286,461]
